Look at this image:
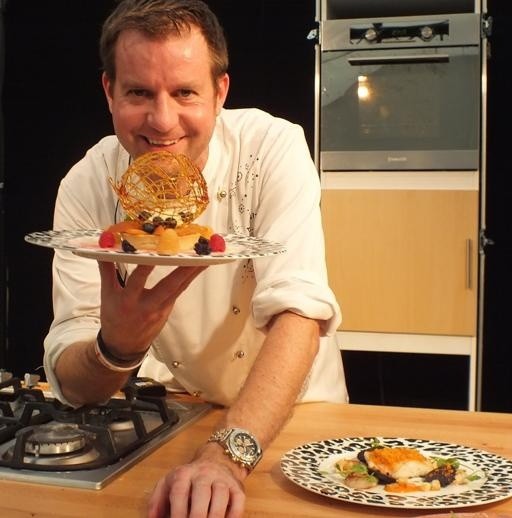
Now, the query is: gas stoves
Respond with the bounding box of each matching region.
[0,367,215,492]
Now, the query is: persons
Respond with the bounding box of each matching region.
[42,0,350,518]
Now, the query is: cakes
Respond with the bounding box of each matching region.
[118,218,212,252]
[371,447,434,477]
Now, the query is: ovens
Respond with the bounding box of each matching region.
[317,16,484,174]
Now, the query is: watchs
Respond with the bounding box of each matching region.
[205,427,263,476]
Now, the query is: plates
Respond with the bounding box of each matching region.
[23,225,289,266]
[278,435,511,510]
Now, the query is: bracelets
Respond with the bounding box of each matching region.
[94,327,151,365]
[92,337,149,372]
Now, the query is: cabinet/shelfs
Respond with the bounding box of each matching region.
[320,188,481,339]
